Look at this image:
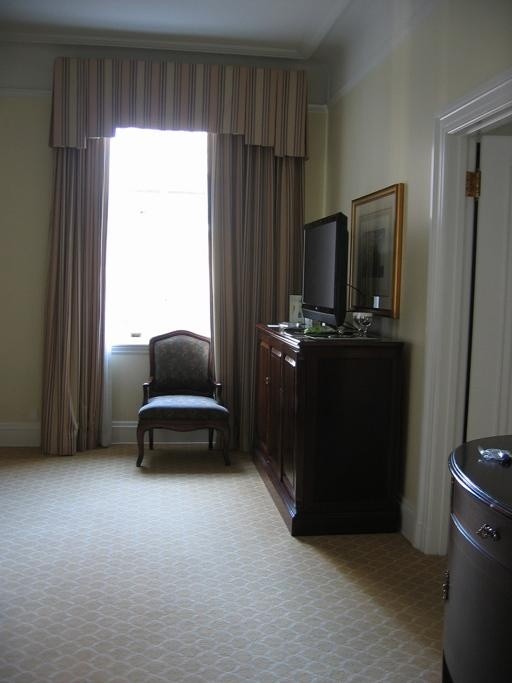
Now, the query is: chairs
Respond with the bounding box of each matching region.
[136,329,231,467]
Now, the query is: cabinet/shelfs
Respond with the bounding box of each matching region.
[252,323,407,536]
[440,434,512,683]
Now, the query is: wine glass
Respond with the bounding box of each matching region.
[360,313,376,337]
[352,312,368,338]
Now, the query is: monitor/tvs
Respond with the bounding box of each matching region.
[301,211,349,328]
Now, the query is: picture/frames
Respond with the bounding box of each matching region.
[349,182,404,320]
